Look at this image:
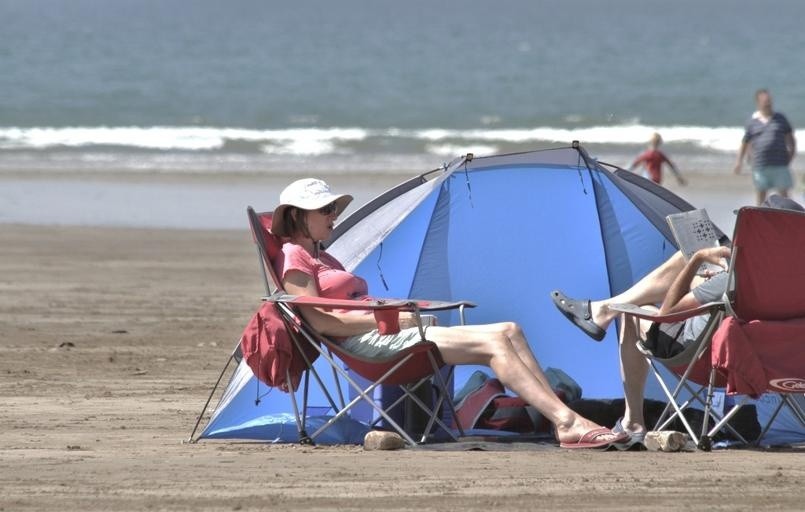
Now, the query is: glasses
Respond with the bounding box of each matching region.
[316,201,338,216]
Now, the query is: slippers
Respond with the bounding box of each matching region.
[550,290,606,342]
[610,414,649,442]
[559,417,632,449]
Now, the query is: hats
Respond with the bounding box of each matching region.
[272,178,354,234]
[732,193,804,216]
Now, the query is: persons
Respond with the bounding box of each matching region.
[272,176,618,452]
[731,86,798,206]
[547,194,805,440]
[622,130,690,191]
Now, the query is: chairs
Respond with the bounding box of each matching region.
[245,203,479,445]
[605,206,804,448]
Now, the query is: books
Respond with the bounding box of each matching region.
[665,206,726,276]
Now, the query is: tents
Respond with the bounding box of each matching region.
[191,139,805,452]
